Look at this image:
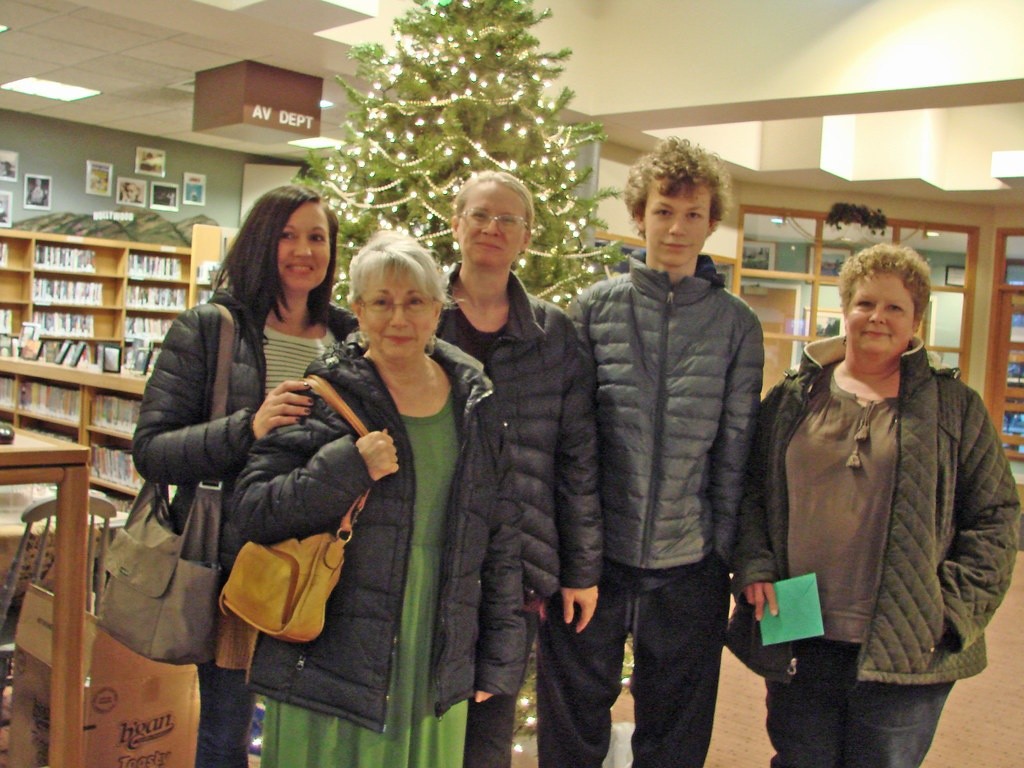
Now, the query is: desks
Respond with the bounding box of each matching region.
[0,421,91,768]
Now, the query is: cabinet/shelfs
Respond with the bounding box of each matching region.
[0,224,241,365]
[0,357,152,497]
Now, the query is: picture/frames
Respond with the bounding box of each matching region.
[116,176,148,208]
[0,191,12,227]
[742,237,779,271]
[914,295,937,347]
[806,245,853,286]
[21,340,152,376]
[23,173,52,211]
[85,160,114,197]
[945,265,965,286]
[182,172,207,206]
[0,150,20,183]
[802,306,846,348]
[134,146,167,178]
[149,180,180,212]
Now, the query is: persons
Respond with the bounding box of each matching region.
[27,178,48,205]
[534,135,765,768]
[729,242,1020,768]
[231,228,527,768]
[435,169,604,768]
[120,182,141,203]
[166,190,175,206]
[131,184,361,768]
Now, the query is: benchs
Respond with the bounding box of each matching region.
[0,492,116,632]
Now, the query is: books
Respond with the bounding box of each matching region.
[89,394,141,435]
[126,316,172,338]
[34,277,103,305]
[35,244,95,272]
[0,377,13,407]
[18,381,80,424]
[1009,311,1024,344]
[90,444,145,489]
[761,573,824,646]
[0,243,8,265]
[0,308,12,333]
[129,254,181,281]
[1002,348,1024,452]
[127,285,186,310]
[34,311,94,337]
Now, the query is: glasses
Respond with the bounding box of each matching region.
[359,295,437,313]
[457,208,531,232]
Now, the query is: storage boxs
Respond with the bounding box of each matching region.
[8,583,200,768]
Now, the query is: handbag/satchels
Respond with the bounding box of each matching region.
[101,478,222,666]
[219,532,347,643]
